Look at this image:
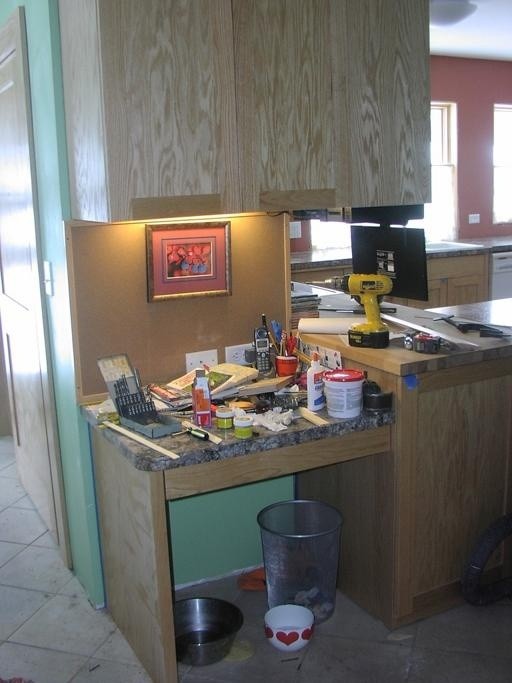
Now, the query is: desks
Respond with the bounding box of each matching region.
[88,387,394,683]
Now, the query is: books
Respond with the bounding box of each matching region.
[167,360,295,402]
[290,289,323,330]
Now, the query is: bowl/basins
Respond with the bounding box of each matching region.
[263,604,316,653]
[175,596,244,667]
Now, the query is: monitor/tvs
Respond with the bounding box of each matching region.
[351,225,428,301]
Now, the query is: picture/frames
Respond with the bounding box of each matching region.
[146,219,233,302]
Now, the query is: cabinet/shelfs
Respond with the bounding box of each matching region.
[296,313,511,630]
[346,0,431,210]
[291,253,489,310]
[56,0,245,225]
[232,0,347,215]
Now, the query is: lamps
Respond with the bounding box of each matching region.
[429,1,479,28]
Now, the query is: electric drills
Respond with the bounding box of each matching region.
[304,273,393,348]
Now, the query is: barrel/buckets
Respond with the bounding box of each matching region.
[322,368,366,420]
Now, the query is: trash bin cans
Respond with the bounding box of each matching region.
[256,499,345,625]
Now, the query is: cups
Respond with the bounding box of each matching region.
[275,354,298,378]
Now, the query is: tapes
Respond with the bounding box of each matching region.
[232,415,254,427]
[216,407,234,418]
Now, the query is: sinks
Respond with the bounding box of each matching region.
[426,239,462,252]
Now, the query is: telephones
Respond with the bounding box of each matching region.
[253,313,276,379]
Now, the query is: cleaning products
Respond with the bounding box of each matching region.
[307,354,325,411]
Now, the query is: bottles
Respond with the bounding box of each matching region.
[306,352,327,410]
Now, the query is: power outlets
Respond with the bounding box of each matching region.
[224,341,254,366]
[185,349,218,373]
